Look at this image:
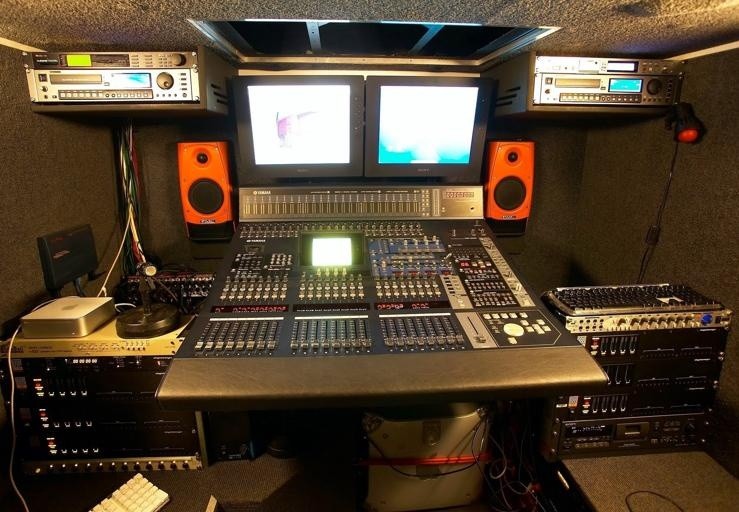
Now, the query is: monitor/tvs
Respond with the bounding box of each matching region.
[365,76,495,180]
[228,75,366,185]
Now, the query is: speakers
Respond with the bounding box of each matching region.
[483,139,537,238]
[176,142,236,242]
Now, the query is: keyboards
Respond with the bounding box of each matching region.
[87,471,171,511]
